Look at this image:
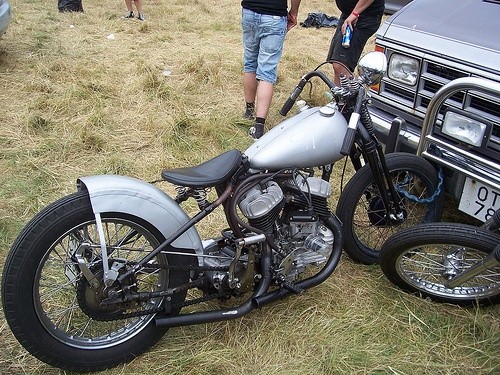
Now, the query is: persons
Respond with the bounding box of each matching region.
[326,0,385,88]
[124,0,144,20]
[241,0,301,142]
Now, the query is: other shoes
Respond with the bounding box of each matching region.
[245,108,256,119]
[248,123,265,141]
[122,10,134,19]
[137,12,144,21]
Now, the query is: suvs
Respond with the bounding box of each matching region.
[362,0,500,223]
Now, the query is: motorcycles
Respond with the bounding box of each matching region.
[1,50,442,374]
[380,208,500,307]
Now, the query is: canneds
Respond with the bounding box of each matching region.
[342,26,353,48]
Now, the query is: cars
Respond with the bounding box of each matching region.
[0,1,13,39]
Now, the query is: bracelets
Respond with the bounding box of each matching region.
[289,11,297,19]
[352,11,360,18]
